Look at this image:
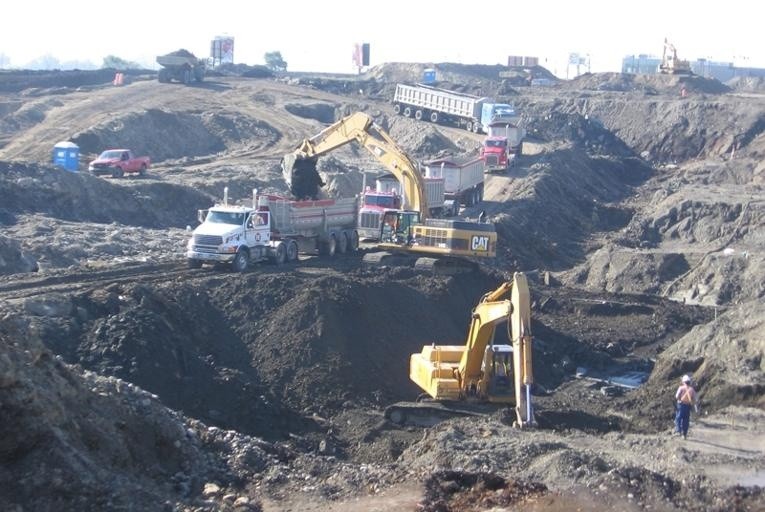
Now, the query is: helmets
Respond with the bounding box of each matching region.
[682,375,692,385]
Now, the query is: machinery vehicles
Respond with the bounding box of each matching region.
[279,111,501,279]
[382,269,545,433]
[656,37,694,76]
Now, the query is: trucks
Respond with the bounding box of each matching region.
[182,184,362,273]
[389,81,514,134]
[499,70,532,86]
[480,112,528,175]
[156,53,207,86]
[360,169,450,244]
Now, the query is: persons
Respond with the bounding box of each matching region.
[676,375,699,440]
[252,213,264,226]
[384,222,393,237]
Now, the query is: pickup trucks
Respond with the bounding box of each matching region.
[87,147,151,180]
